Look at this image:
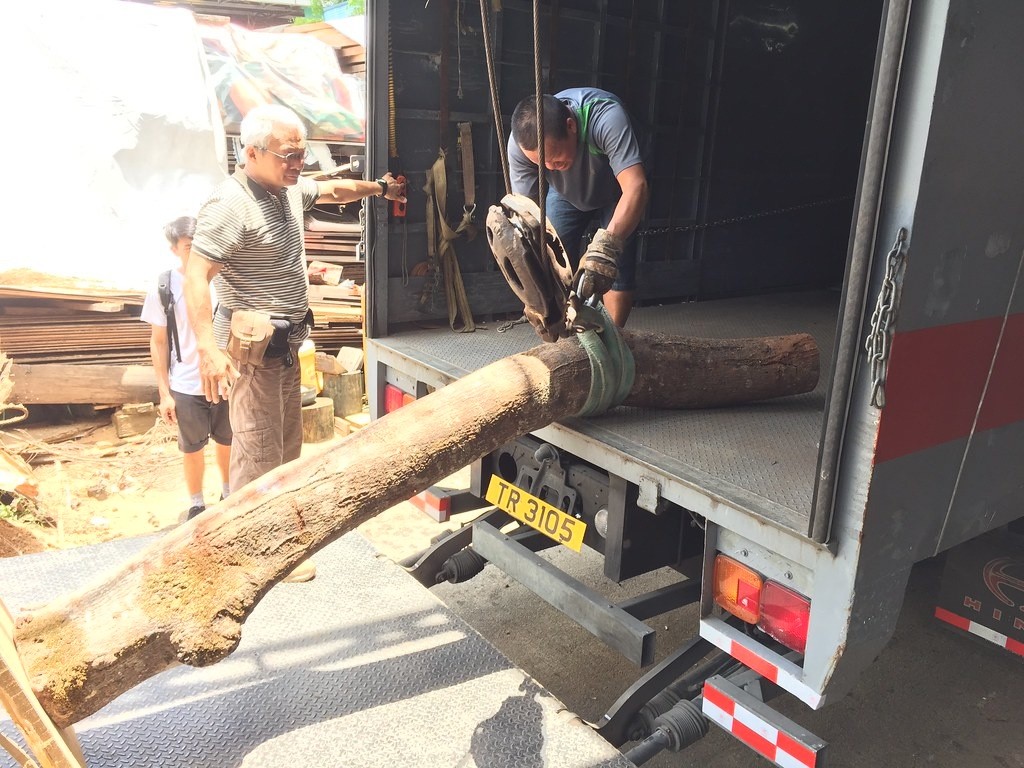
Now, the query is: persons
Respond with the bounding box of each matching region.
[140,217,234,520]
[183,104,407,584]
[508,87,650,328]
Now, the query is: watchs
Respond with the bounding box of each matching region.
[374,178,388,198]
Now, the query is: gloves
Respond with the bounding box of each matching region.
[572,228,624,298]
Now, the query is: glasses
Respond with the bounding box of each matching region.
[257,146,309,162]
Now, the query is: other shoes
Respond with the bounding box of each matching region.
[220,493,224,501]
[282,559,316,583]
[187,506,206,521]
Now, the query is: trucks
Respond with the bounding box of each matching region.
[364,0,1023,765]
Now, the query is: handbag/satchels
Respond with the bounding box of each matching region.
[226,309,292,365]
